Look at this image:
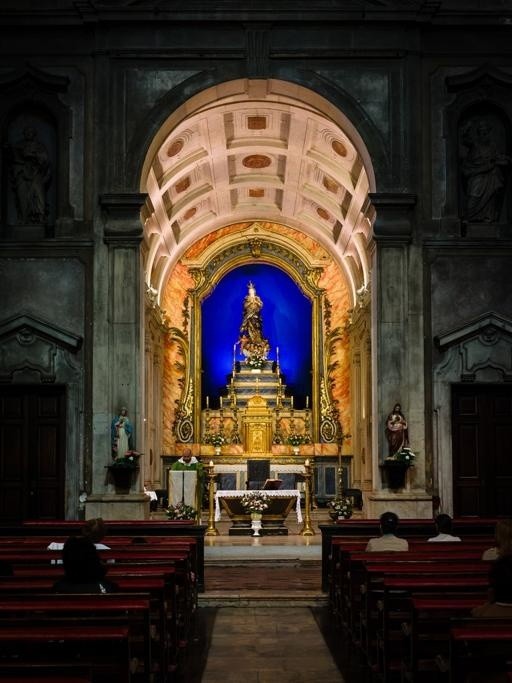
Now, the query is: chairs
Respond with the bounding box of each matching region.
[245,460,270,490]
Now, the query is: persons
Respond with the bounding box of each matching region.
[48,517,115,565]
[172,447,205,508]
[468,518,511,619]
[49,536,125,595]
[479,522,501,560]
[427,513,461,540]
[384,402,409,460]
[111,406,136,461]
[365,510,408,551]
[235,279,270,358]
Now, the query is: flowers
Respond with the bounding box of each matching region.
[165,503,197,520]
[327,497,355,515]
[110,447,142,465]
[287,435,305,446]
[395,448,415,462]
[240,491,272,513]
[210,433,225,446]
[244,349,268,369]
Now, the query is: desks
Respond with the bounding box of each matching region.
[214,490,303,535]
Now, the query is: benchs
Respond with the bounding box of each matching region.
[319,518,512,683]
[0,520,209,683]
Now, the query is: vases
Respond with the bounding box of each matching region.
[109,465,136,493]
[251,513,263,536]
[293,446,299,455]
[384,459,410,491]
[251,369,261,374]
[329,510,353,520]
[215,447,222,456]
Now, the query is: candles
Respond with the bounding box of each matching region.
[279,378,281,384]
[276,396,279,408]
[305,458,308,466]
[277,346,280,365]
[206,396,209,409]
[291,396,294,409]
[233,394,237,408]
[306,396,309,408]
[231,378,234,384]
[234,344,236,361]
[220,396,222,407]
[209,460,213,465]
